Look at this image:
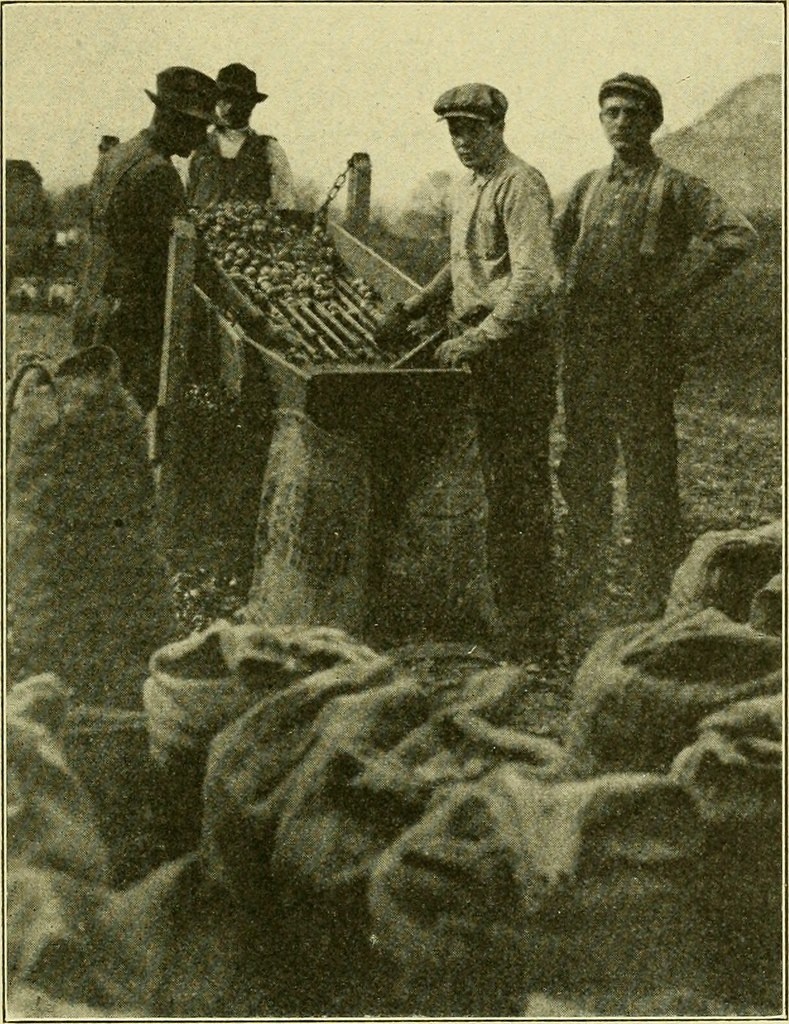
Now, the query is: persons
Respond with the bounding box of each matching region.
[185,63,295,209]
[68,66,231,412]
[374,83,557,663]
[552,73,760,545]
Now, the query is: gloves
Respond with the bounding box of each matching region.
[373,302,415,351]
[433,327,489,368]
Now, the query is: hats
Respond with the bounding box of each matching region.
[144,67,231,127]
[216,64,268,103]
[434,83,508,122]
[599,73,663,122]
